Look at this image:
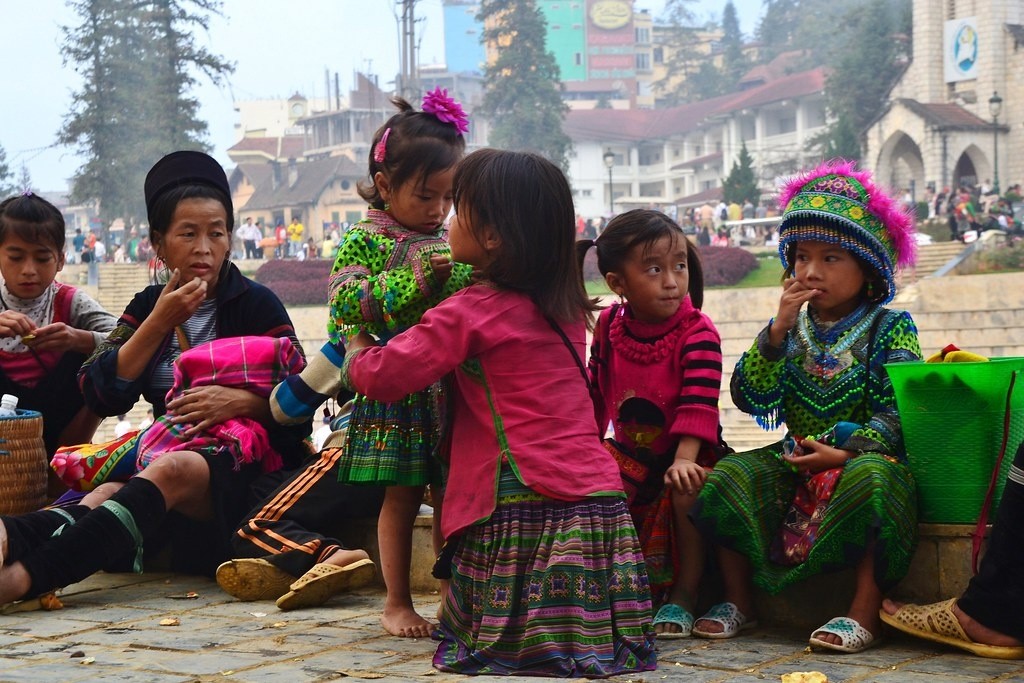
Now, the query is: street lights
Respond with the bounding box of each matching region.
[603,146,615,213]
[987,90,1003,195]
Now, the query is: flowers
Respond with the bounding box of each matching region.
[421,86,469,136]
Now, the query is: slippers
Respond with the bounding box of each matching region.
[809,614,878,652]
[691,602,757,639]
[276,557,376,612]
[216,556,300,600]
[878,595,1024,660]
[651,603,695,637]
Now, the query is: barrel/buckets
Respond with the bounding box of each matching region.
[885,357,1024,523]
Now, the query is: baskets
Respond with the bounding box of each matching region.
[0,409,49,514]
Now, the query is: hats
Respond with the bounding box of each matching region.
[144,150,232,211]
[776,165,909,306]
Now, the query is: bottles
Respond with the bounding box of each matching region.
[0,393,18,416]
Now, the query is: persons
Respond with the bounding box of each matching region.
[216,331,386,611]
[0,190,121,449]
[879,438,1024,659]
[237,216,335,259]
[923,177,1024,232]
[327,87,475,638]
[904,188,912,202]
[577,214,607,240]
[65,227,151,264]
[669,198,780,248]
[0,150,314,608]
[686,163,925,653]
[577,208,735,638]
[342,148,659,677]
